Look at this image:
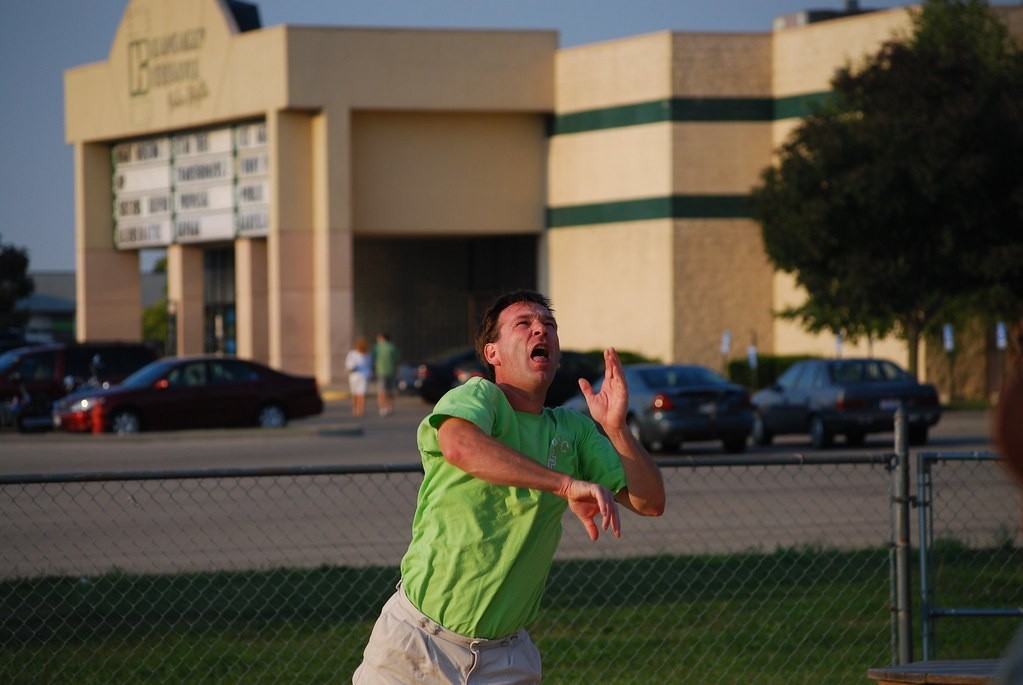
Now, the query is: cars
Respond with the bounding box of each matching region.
[751,357,942,449]
[556,362,753,456]
[0,339,157,427]
[386,343,479,401]
[49,355,325,435]
[448,346,653,405]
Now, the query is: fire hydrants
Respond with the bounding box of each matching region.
[90,400,104,434]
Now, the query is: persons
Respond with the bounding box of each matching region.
[8,371,30,432]
[87,361,111,391]
[351,290,665,685]
[372,329,400,419]
[65,374,85,393]
[345,334,373,417]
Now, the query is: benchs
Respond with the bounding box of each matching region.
[867,658,1009,685]
